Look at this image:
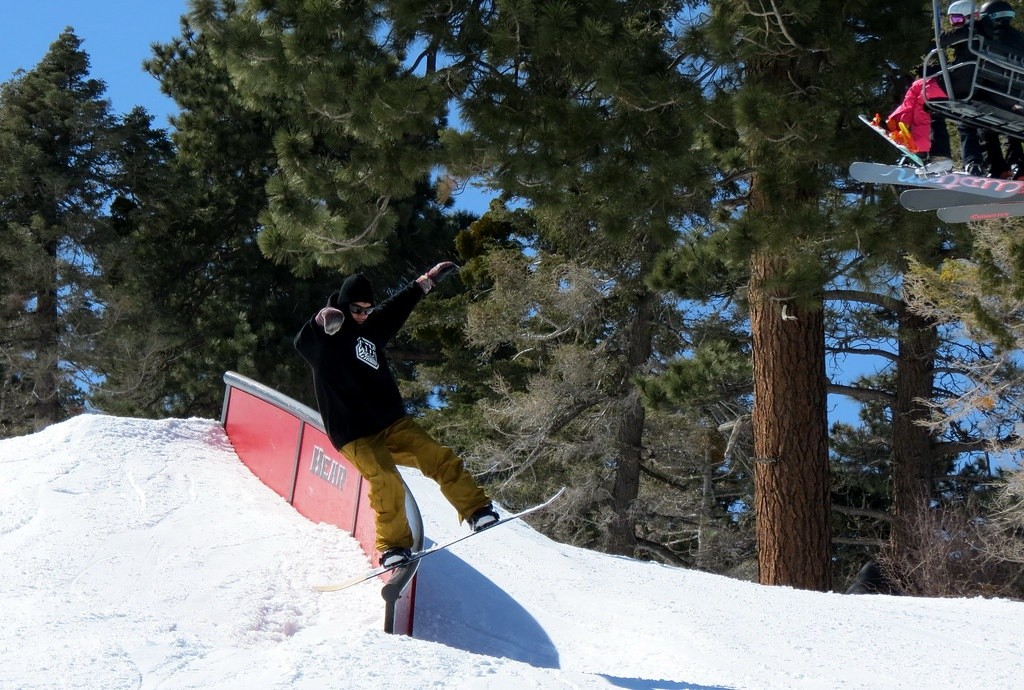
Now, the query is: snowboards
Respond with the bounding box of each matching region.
[308,487,567,595]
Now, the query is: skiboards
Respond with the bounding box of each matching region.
[899,189,1024,224]
[847,113,1024,199]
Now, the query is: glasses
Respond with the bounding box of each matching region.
[348,304,375,315]
[948,15,966,26]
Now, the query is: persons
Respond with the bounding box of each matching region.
[297,261,499,573]
[884,0,1024,178]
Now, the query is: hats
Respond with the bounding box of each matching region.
[338,274,374,306]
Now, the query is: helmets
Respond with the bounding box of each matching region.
[946,0,977,18]
[975,0,1016,22]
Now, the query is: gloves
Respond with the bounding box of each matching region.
[913,65,940,78]
[416,261,460,295]
[929,35,944,60]
[315,307,344,336]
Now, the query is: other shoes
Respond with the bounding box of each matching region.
[963,157,985,176]
[987,160,1024,180]
[378,546,411,569]
[467,503,499,532]
[914,158,954,178]
